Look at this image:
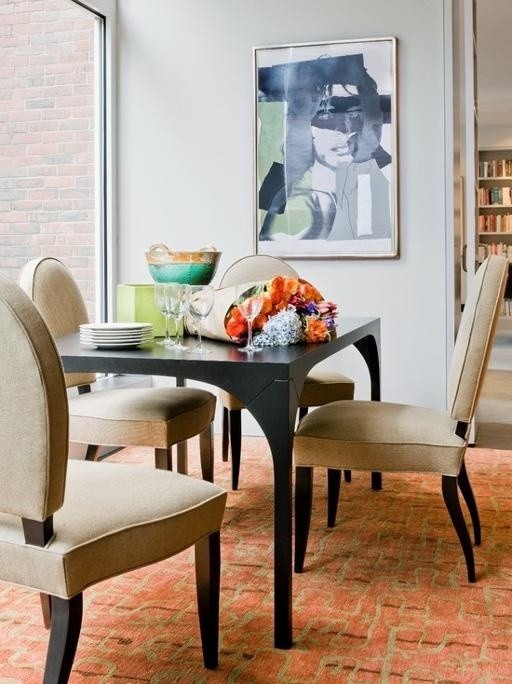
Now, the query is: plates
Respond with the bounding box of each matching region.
[79,323,154,352]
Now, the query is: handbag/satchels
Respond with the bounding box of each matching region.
[183,276,336,344]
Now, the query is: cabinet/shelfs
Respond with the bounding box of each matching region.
[477,147,512,265]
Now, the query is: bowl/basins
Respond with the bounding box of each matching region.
[144,244,222,285]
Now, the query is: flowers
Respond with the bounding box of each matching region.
[223,277,338,346]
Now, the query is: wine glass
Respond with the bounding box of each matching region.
[153,283,214,354]
[237,284,263,352]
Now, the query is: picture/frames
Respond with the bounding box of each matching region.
[253,36,399,261]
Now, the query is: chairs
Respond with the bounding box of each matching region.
[293,255,509,582]
[20,257,216,482]
[218,255,356,491]
[0,271,228,684]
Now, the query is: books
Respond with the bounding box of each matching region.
[477,158,512,318]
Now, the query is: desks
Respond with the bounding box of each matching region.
[57,316,382,649]
